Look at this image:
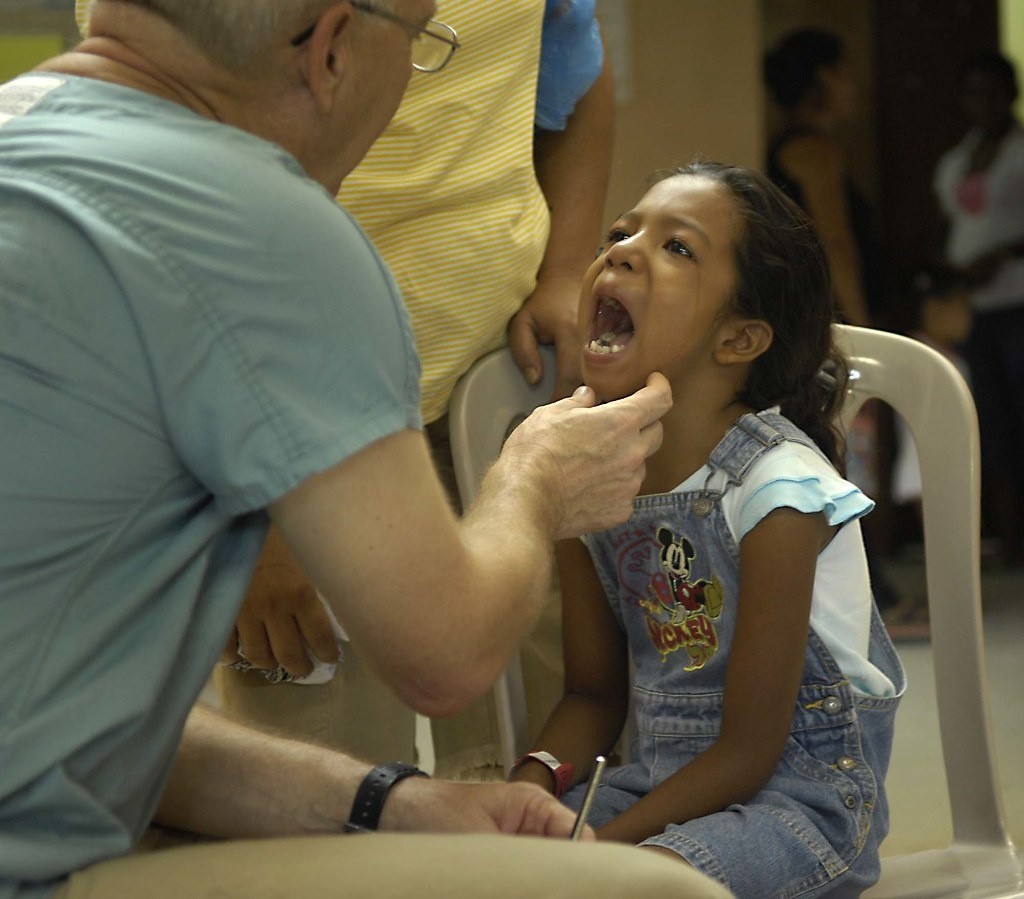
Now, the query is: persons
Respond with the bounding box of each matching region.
[505,160,904,899]
[68,0,1024,791]
[0,0,731,899]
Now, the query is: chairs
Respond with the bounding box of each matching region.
[445,322,1024,899]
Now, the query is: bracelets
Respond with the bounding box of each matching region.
[507,752,576,797]
[343,759,429,834]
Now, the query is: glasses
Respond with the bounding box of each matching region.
[292,0,461,74]
